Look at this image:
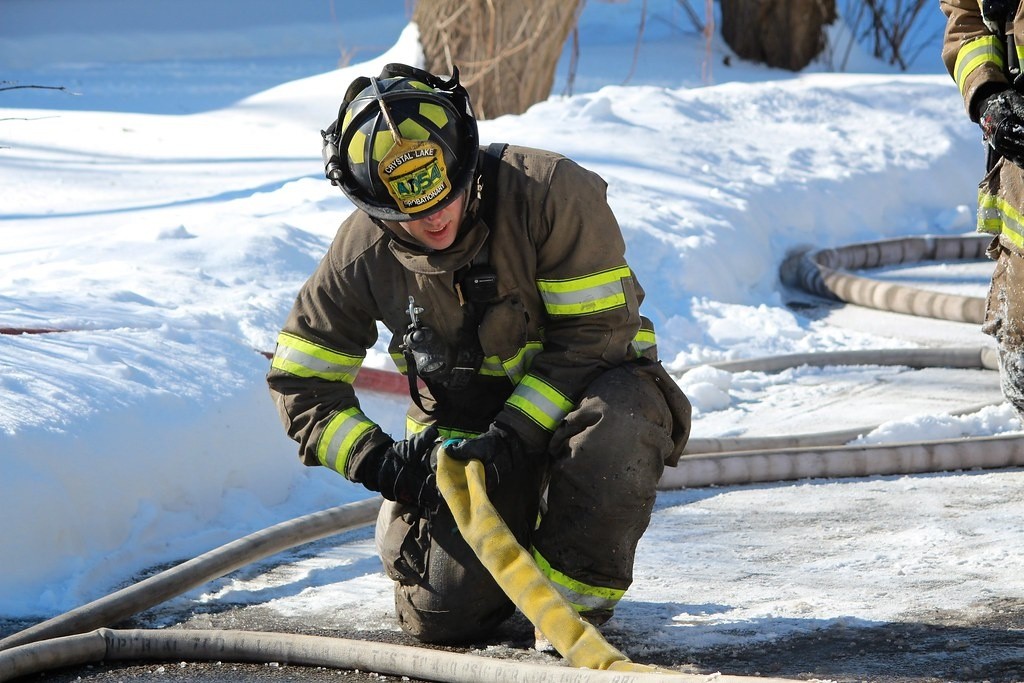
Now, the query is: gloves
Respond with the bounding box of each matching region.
[974,88,1024,170]
[446,417,526,499]
[380,421,461,527]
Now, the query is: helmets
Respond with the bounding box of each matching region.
[322,72,480,222]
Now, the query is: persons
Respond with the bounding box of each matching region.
[268,66,692,652]
[939,0,1024,419]
